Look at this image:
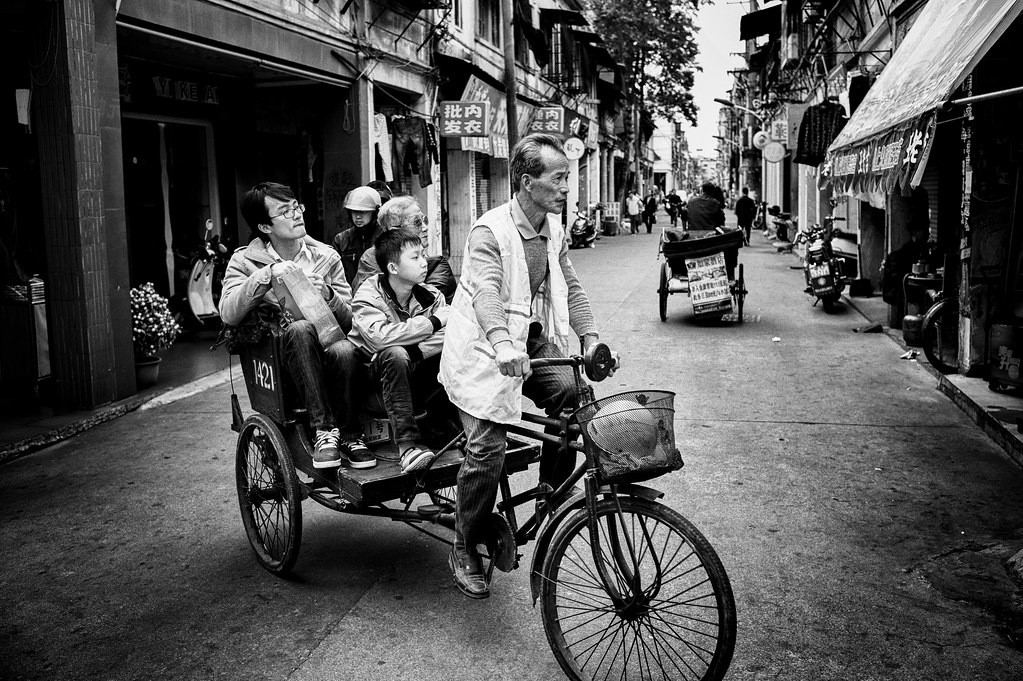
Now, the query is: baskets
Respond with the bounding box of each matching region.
[572,390,676,480]
[175,248,190,277]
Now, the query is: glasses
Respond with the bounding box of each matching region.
[270,204,305,219]
[392,216,429,228]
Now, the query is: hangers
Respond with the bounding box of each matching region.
[819,97,831,106]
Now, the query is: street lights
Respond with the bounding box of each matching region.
[712,136,742,196]
[714,97,766,208]
[715,148,732,209]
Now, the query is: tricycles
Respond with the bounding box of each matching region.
[228,318,737,681]
[656,225,749,323]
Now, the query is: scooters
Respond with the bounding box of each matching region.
[167,218,242,351]
[570,202,602,249]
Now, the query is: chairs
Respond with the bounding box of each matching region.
[922,252,962,361]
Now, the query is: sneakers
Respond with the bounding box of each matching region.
[338,436,377,468]
[312,428,341,468]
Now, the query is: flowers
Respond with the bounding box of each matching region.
[128,283,183,362]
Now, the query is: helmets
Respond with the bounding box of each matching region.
[344,186,382,212]
[587,400,667,466]
[366,180,394,201]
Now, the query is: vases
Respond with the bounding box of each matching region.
[134,355,162,387]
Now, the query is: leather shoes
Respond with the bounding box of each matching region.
[448,552,489,599]
[537,485,597,509]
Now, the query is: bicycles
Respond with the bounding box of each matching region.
[670,203,681,227]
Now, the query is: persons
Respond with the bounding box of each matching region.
[734,187,756,246]
[684,182,726,230]
[217,178,457,474]
[436,132,622,601]
[626,187,683,235]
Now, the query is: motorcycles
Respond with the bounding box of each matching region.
[792,216,846,310]
[751,203,764,229]
[768,204,790,240]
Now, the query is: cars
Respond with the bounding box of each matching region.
[665,189,692,215]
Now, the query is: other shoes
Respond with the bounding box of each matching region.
[398,447,434,474]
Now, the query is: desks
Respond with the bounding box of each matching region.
[903,271,943,317]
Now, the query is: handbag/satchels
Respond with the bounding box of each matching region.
[651,214,656,224]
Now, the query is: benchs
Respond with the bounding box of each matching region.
[659,228,742,276]
[220,255,338,424]
